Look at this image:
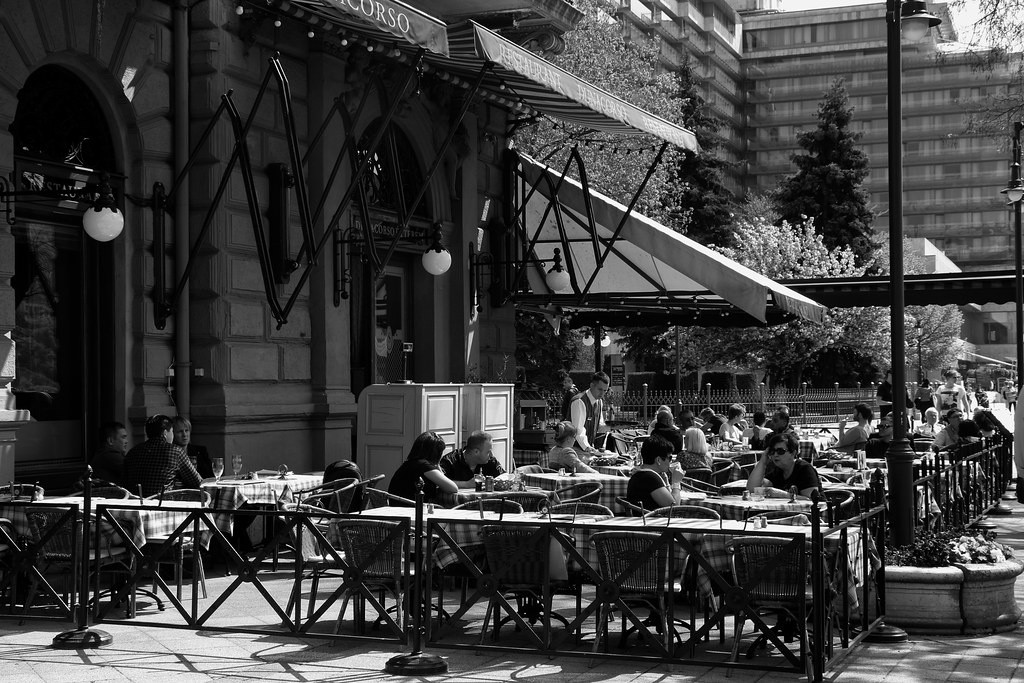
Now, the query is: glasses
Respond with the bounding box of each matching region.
[769,448,789,455]
[953,376,959,379]
[668,456,672,462]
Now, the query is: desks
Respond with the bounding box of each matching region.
[812,465,885,510]
[662,491,823,609]
[1,492,214,611]
[522,471,634,571]
[706,448,765,484]
[723,479,868,521]
[669,521,882,656]
[422,511,612,644]
[827,452,938,483]
[572,517,737,649]
[342,506,496,638]
[590,459,677,476]
[202,470,332,562]
[798,433,829,458]
[425,482,548,588]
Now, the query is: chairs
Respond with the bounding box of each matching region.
[1,429,984,683]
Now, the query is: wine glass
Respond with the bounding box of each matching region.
[629,447,637,467]
[212,458,224,483]
[231,454,243,479]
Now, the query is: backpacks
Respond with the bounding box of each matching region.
[318,459,365,512]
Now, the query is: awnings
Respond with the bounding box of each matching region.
[399,18,703,155]
[291,0,450,58]
[511,154,828,323]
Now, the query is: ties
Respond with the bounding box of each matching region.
[593,403,597,415]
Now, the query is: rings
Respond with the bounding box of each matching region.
[768,494,771,497]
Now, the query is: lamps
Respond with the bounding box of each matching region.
[0,172,134,242]
[469,242,567,318]
[334,218,451,307]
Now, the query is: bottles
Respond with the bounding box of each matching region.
[247,471,258,480]
[509,481,524,491]
[558,468,565,476]
[833,463,842,472]
[423,503,428,514]
[485,476,494,492]
[754,517,761,529]
[761,517,767,528]
[428,504,435,514]
[742,491,749,500]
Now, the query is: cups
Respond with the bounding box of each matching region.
[475,481,483,491]
[669,462,681,473]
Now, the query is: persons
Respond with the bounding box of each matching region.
[124,413,205,500]
[440,433,506,488]
[172,416,214,479]
[388,431,457,507]
[549,372,1024,517]
[92,421,127,483]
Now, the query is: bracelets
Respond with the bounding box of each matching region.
[671,483,681,490]
